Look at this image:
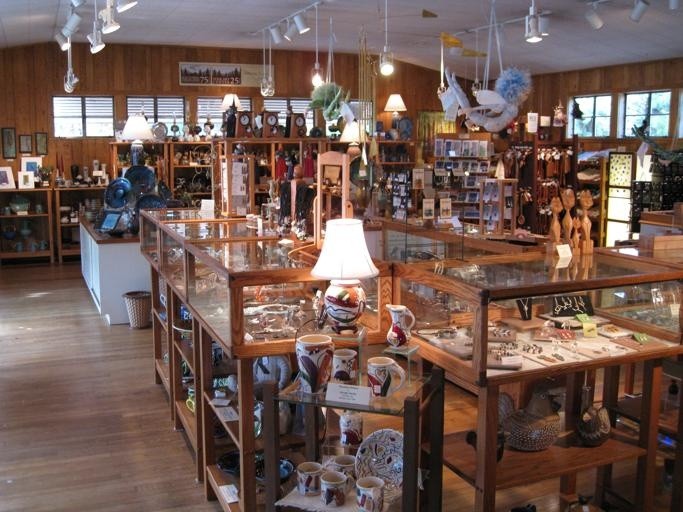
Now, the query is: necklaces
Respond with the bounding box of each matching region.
[551,295,587,315]
[520,298,529,319]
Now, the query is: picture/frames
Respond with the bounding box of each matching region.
[435,139,490,219]
[606,152,636,247]
[0,127,47,189]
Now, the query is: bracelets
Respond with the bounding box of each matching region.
[486,338,543,364]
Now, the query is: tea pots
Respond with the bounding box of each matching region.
[385,304,416,348]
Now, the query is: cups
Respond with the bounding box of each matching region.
[332,349,356,383]
[295,462,321,496]
[319,469,347,506]
[295,334,335,395]
[257,303,294,332]
[332,454,354,490]
[354,477,384,511]
[367,356,406,399]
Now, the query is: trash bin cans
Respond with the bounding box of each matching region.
[122,291,152,329]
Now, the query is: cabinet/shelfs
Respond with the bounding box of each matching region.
[54,186,107,263]
[109,137,414,216]
[0,189,53,263]
[140,216,683,512]
[578,160,601,221]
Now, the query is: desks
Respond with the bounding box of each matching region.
[78,216,152,325]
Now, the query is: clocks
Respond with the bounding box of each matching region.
[235,111,305,137]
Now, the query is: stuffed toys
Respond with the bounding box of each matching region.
[305,80,354,122]
[444,67,533,133]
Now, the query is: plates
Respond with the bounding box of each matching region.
[104,177,132,209]
[134,195,164,216]
[123,166,154,194]
[354,428,403,489]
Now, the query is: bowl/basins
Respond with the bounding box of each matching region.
[58,196,104,225]
[172,318,192,339]
[217,449,295,487]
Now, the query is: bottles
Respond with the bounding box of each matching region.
[338,410,363,449]
[0,196,48,252]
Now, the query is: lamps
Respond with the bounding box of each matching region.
[252,0,324,96]
[221,94,243,137]
[669,0,679,10]
[384,94,407,129]
[584,0,607,31]
[436,10,551,98]
[553,98,567,127]
[525,0,542,43]
[378,0,394,75]
[121,115,152,166]
[54,0,138,93]
[629,0,649,23]
